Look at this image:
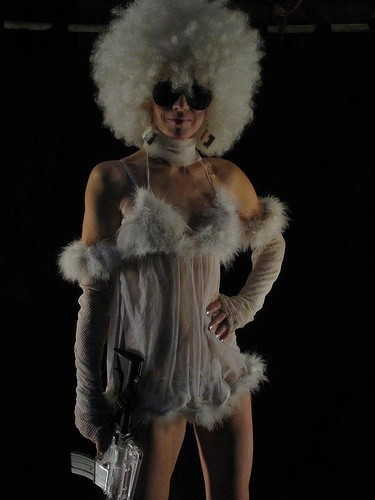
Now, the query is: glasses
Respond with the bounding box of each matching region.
[153,80,212,110]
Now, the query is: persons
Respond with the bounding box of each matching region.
[57,1,288,500]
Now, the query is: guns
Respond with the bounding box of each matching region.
[70,349,144,500]
